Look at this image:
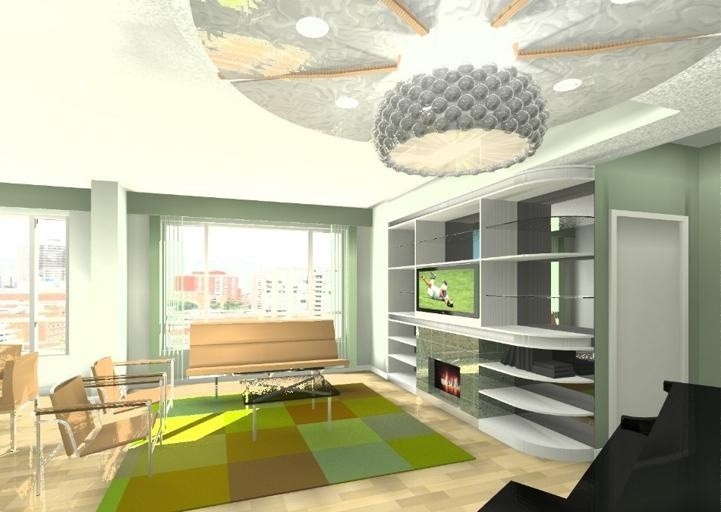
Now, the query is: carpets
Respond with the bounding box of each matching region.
[97,382,476,512]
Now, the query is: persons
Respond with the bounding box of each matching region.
[422,273,454,307]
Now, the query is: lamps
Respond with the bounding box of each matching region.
[369,7,552,180]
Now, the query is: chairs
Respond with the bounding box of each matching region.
[186,317,350,403]
[0,344,174,496]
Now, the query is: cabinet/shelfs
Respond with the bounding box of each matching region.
[386,164,596,464]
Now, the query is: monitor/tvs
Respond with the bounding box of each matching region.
[417,263,479,318]
